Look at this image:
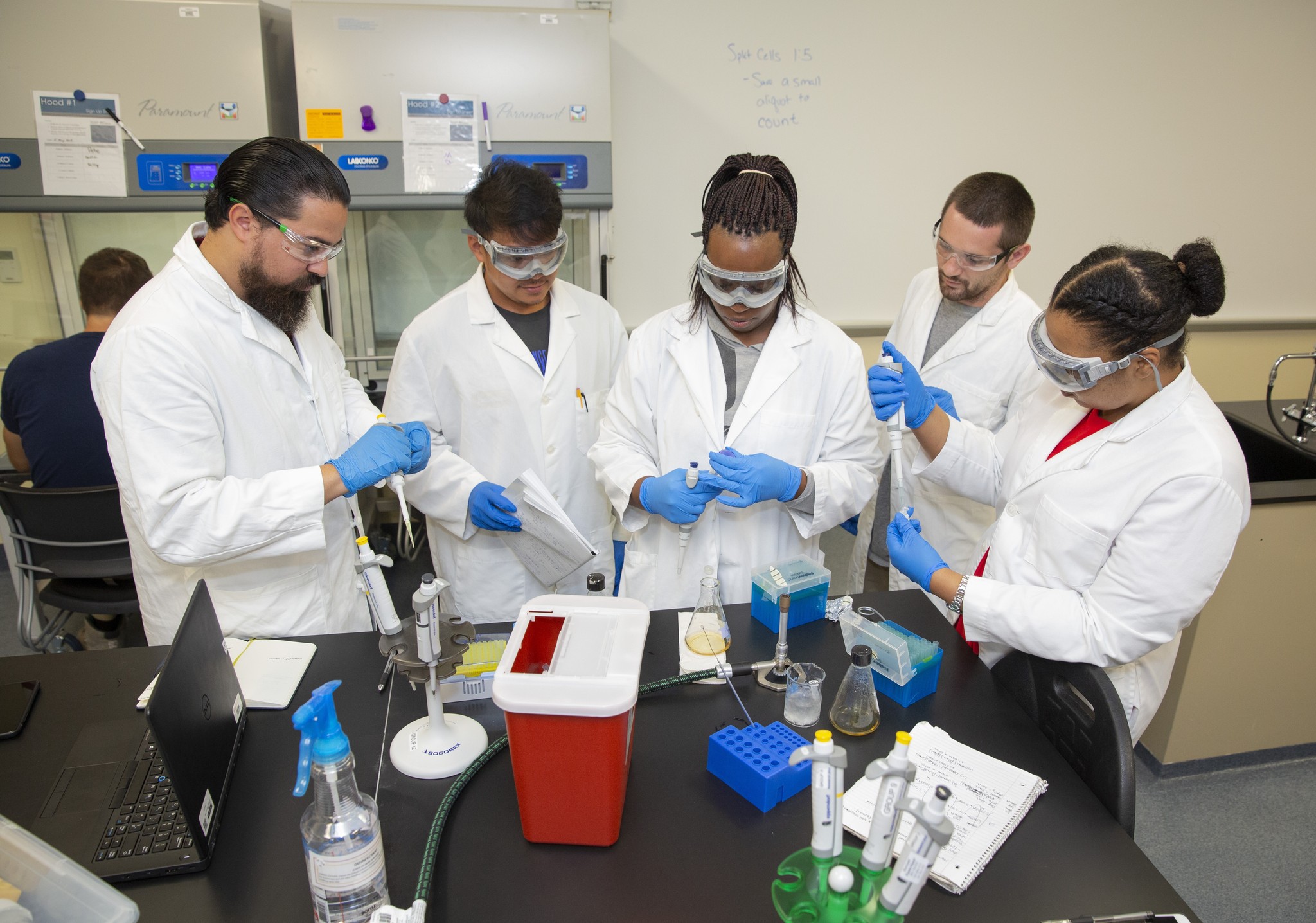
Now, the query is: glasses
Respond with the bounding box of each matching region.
[1027,308,1186,390]
[931,216,1023,273]
[461,224,569,281]
[228,195,346,266]
[696,254,790,309]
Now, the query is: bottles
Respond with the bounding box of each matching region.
[586,572,607,596]
[685,577,732,656]
[828,644,881,736]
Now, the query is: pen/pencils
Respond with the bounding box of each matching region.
[378,650,397,691]
[106,107,145,151]
[576,387,583,408]
[581,393,589,413]
[1039,911,1155,923]
[483,102,491,152]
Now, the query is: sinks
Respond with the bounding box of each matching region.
[1222,410,1316,483]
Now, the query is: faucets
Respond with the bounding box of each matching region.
[1265,350,1316,442]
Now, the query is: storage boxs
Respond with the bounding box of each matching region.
[746,552,835,634]
[492,593,655,853]
[832,607,947,708]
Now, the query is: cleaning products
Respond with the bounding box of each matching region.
[288,681,392,923]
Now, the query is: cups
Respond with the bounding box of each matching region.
[783,662,826,728]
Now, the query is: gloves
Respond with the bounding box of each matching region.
[924,386,961,423]
[886,507,950,595]
[867,340,935,429]
[709,446,802,509]
[323,425,412,499]
[389,421,431,474]
[467,482,522,533]
[639,468,724,525]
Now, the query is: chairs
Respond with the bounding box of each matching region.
[1013,659,1137,839]
[0,476,140,655]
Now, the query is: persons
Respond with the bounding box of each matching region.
[868,237,1251,748]
[588,154,829,600]
[0,248,153,632]
[838,172,1042,617]
[90,136,430,647]
[383,155,629,625]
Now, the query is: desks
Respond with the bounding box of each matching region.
[0,601,1203,923]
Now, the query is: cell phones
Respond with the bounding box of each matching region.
[0,681,40,738]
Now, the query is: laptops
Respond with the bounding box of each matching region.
[87,579,246,887]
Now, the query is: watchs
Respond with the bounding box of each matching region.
[944,574,969,614]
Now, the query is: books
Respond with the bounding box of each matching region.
[136,637,317,709]
[494,468,601,589]
[842,721,1048,895]
